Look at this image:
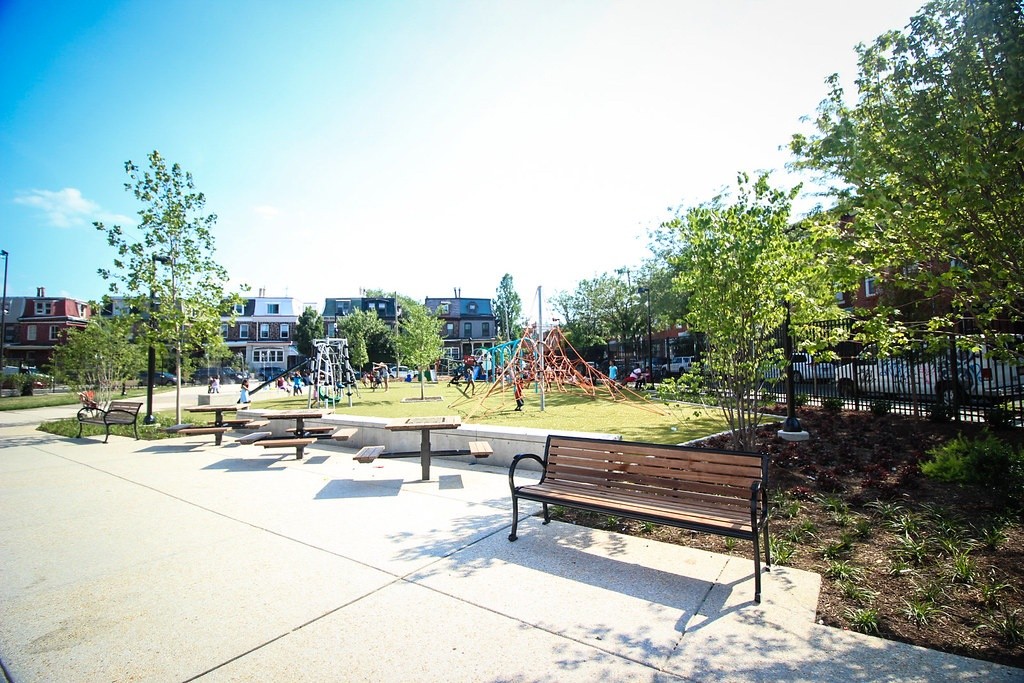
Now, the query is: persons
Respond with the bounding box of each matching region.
[632,366,649,391]
[363,362,389,392]
[241,379,250,403]
[621,363,641,389]
[208,375,220,393]
[608,359,618,393]
[278,371,309,396]
[514,378,524,411]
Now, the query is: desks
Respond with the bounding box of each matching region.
[184,403,251,446]
[261,408,335,460]
[386,416,462,480]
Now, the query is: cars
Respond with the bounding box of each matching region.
[759,351,835,385]
[556,355,694,378]
[189,365,296,385]
[138,371,177,387]
[388,365,414,379]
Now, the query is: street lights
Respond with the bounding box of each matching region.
[142,253,172,425]
[637,287,654,385]
[335,312,346,382]
[0,249,11,370]
[553,318,562,363]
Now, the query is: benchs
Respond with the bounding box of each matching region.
[508,434,771,603]
[76,401,143,444]
[164,418,493,463]
[633,373,650,391]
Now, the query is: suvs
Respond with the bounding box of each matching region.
[834,335,1024,410]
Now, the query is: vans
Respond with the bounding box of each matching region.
[3,364,54,388]
